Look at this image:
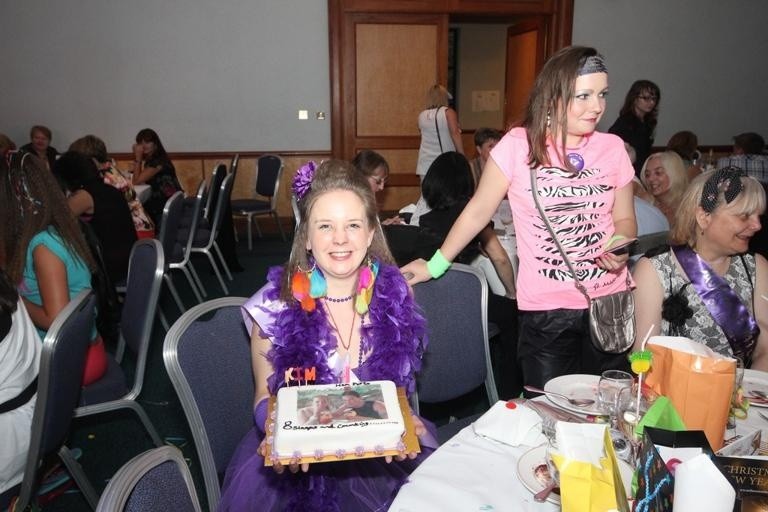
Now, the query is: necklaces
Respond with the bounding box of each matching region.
[323,298,357,356]
[320,292,356,301]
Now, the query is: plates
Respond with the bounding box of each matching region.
[736,368,768,408]
[517,443,636,505]
[544,374,629,415]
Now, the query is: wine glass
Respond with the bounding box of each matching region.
[597,370,635,438]
[718,354,743,402]
[615,387,657,469]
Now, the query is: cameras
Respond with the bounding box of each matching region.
[610,429,631,462]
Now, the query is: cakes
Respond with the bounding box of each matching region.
[268,367,407,456]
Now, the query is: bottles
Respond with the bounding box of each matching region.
[706,148,717,171]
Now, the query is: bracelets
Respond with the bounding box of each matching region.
[134,159,143,165]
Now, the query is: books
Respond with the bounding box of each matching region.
[713,429,768,499]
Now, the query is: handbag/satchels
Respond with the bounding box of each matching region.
[552,420,629,512]
[641,324,737,451]
[632,426,742,512]
[531,162,636,355]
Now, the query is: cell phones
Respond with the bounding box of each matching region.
[593,240,639,262]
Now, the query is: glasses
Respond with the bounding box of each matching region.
[369,175,389,185]
[639,96,661,102]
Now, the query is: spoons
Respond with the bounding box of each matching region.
[612,439,626,452]
[535,481,557,502]
[523,386,595,409]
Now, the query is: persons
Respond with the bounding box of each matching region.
[415,84,465,194]
[0,273,47,509]
[69,134,156,240]
[641,151,689,215]
[623,131,644,175]
[214,158,439,512]
[468,129,502,192]
[352,150,404,225]
[19,125,60,164]
[716,134,767,183]
[0,151,108,386]
[399,46,637,400]
[419,151,516,332]
[609,80,661,159]
[629,166,768,371]
[667,131,703,181]
[131,129,184,216]
[333,390,387,421]
[297,396,333,429]
[51,149,141,281]
[1,133,12,154]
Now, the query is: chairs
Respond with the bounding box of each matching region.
[169,180,207,303]
[179,173,234,294]
[96,445,202,512]
[231,154,288,249]
[115,190,186,334]
[178,164,227,228]
[74,238,176,446]
[185,154,239,208]
[398,263,500,445]
[12,288,101,512]
[163,297,255,512]
